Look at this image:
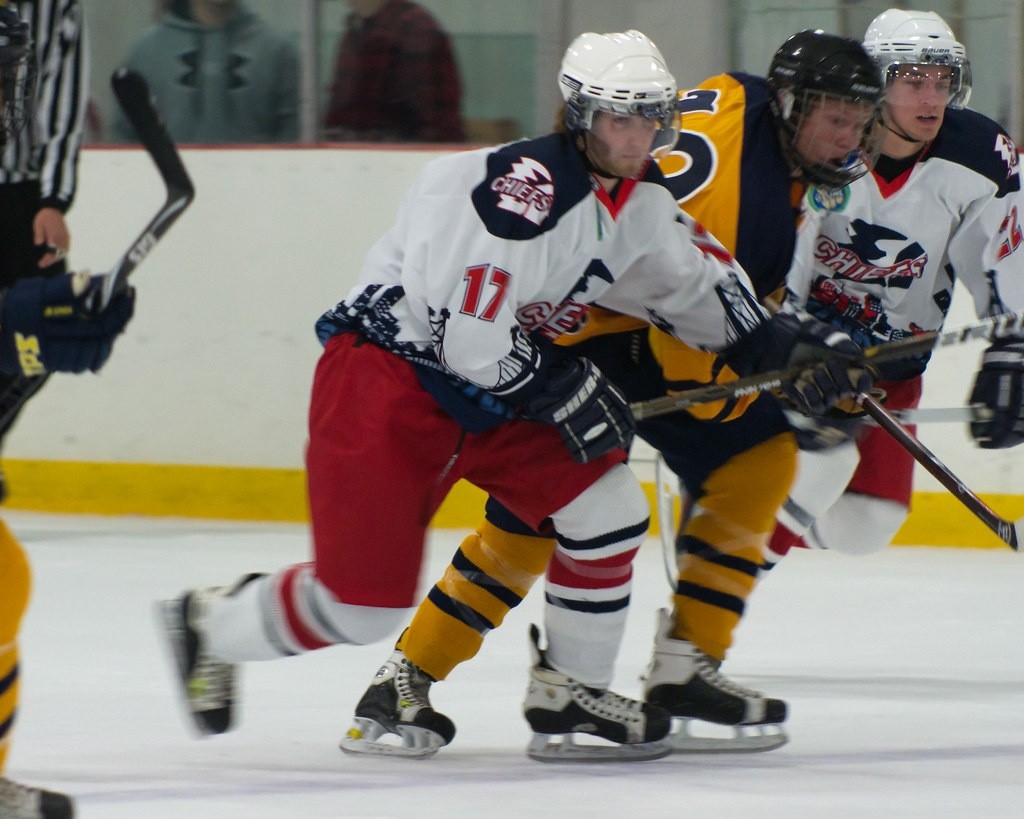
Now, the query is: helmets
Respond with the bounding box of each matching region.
[558,29,683,159]
[860,9,974,111]
[765,29,888,192]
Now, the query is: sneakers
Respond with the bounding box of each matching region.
[154,572,267,739]
[339,627,456,758]
[639,608,789,753]
[522,622,674,764]
[653,452,696,593]
[1,776,74,819]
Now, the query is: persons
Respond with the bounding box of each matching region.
[634,7,1024,683]
[81,0,303,146]
[0,269,136,819]
[0,0,92,507]
[318,1,469,146]
[333,28,892,759]
[153,27,882,740]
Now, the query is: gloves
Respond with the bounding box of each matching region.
[35,271,137,377]
[824,330,865,355]
[522,355,636,465]
[967,336,1024,449]
[786,403,862,451]
[759,330,880,416]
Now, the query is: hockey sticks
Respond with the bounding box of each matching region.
[2,68,196,438]
[852,389,1023,555]
[622,312,1021,422]
